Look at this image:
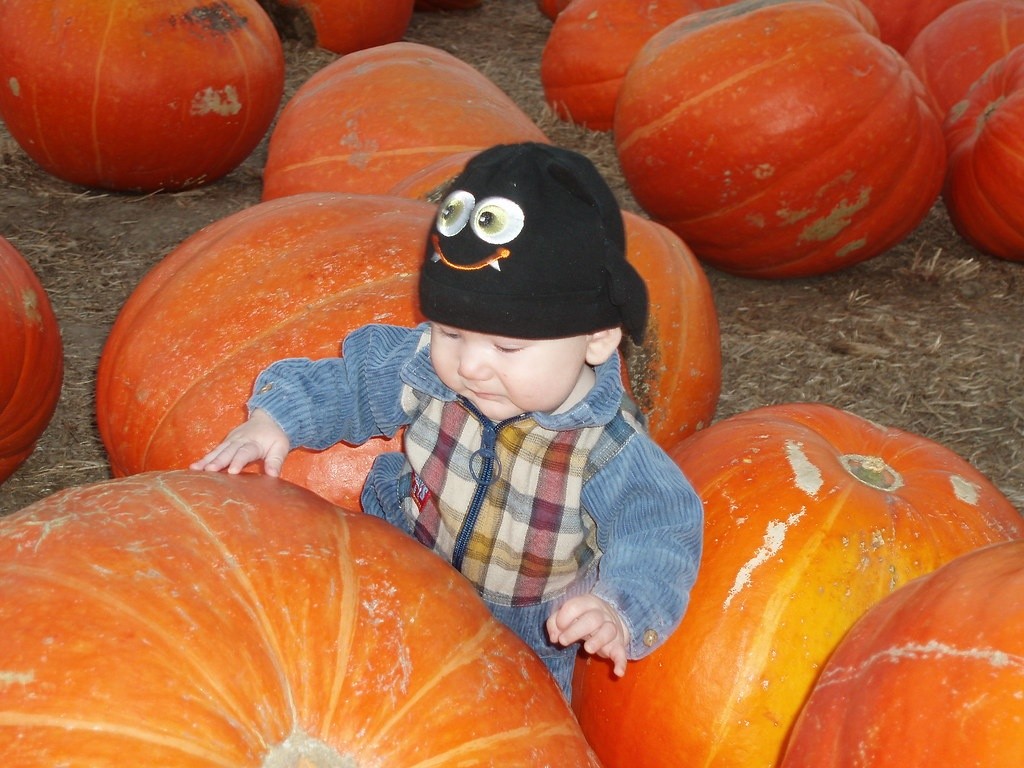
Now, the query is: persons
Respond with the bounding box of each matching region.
[191,142,703,700]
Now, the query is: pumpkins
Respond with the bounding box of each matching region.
[0,0,1024,768]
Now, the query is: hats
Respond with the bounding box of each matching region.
[418,141,651,347]
